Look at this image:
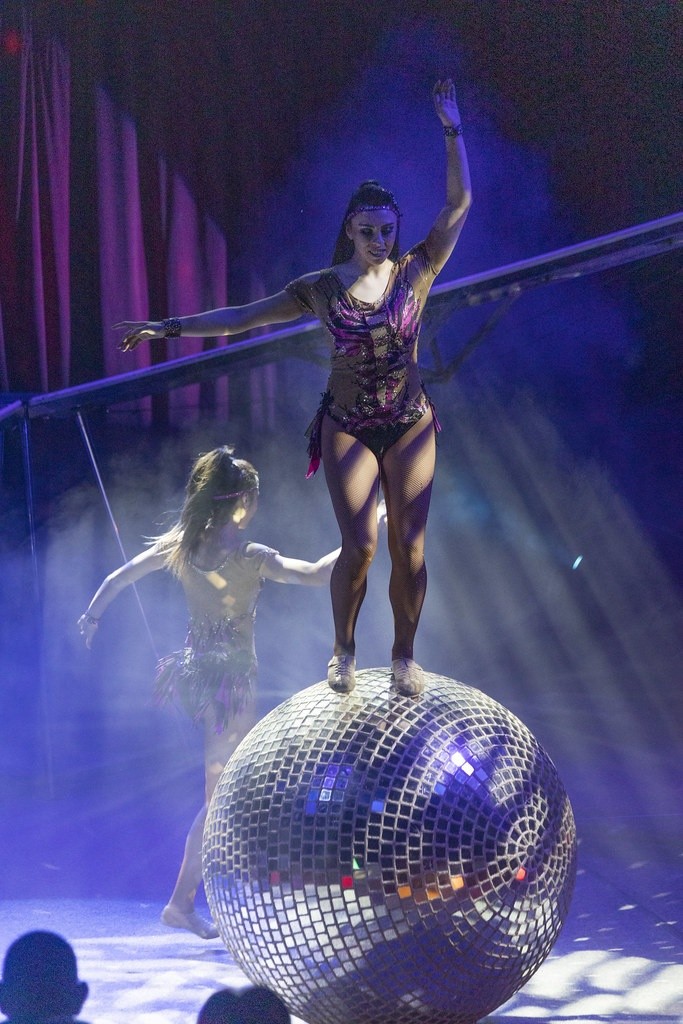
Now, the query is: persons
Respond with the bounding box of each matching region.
[0,929,94,1024]
[197,986,291,1024]
[77,448,387,939]
[110,79,474,697]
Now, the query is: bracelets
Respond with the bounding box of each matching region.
[161,317,181,340]
[442,123,465,138]
[81,610,100,625]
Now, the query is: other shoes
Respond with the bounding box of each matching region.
[160,905,219,939]
[392,657,425,695]
[327,655,355,693]
